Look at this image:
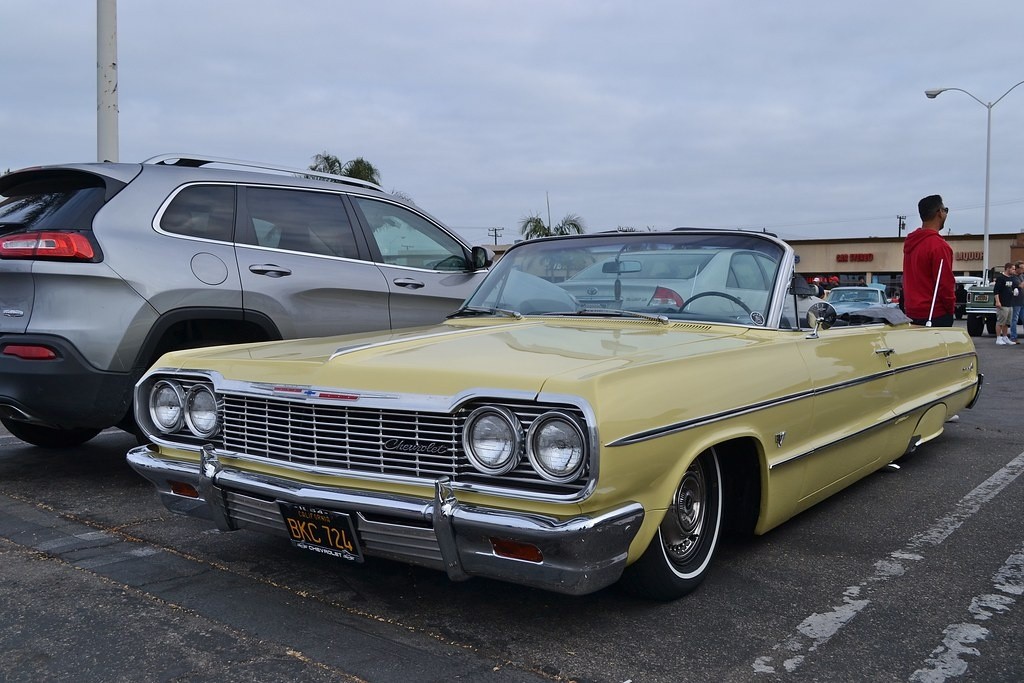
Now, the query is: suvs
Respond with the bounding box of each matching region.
[0,152,581,453]
[967,264,1022,338]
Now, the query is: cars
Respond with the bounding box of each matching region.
[954,275,984,320]
[126,223,970,594]
[825,285,900,316]
[555,247,833,326]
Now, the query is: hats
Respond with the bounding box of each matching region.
[813,278,820,282]
[830,276,839,283]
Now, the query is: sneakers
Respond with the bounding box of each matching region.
[995,336,1006,345]
[1002,335,1016,345]
[1011,338,1020,344]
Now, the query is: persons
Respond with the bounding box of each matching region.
[858,193,1023,346]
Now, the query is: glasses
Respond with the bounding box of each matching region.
[936,207,949,214]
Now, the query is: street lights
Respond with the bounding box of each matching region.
[923,79,1024,287]
